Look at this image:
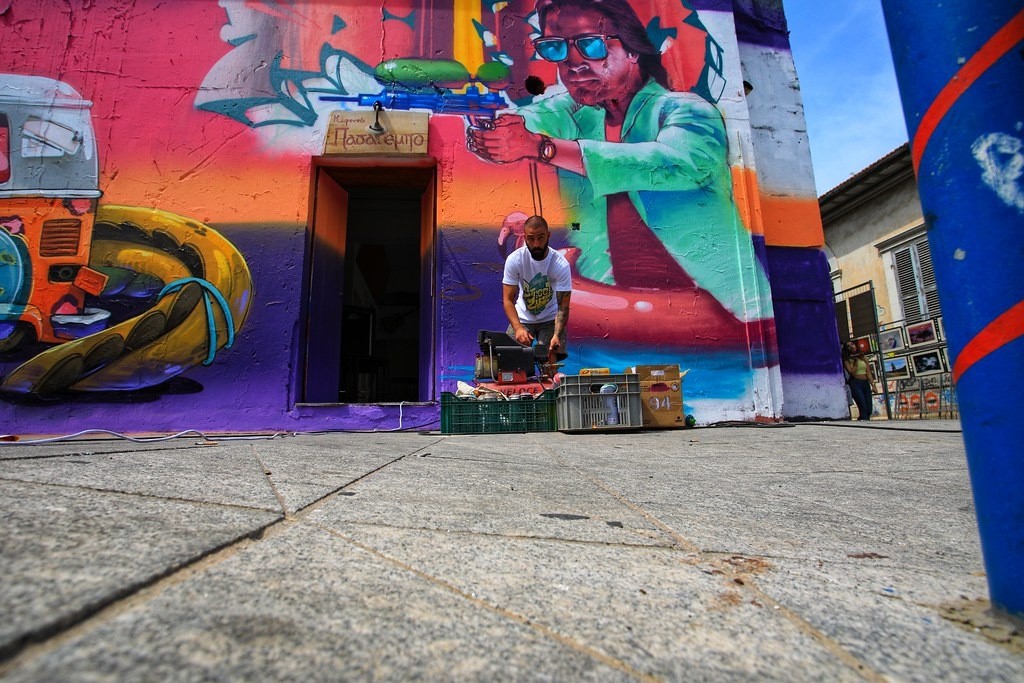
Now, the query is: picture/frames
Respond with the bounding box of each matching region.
[849,315,958,418]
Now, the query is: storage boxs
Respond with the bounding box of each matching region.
[439,363,692,436]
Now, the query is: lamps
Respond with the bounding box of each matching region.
[363,101,388,137]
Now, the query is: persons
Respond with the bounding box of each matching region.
[841,340,879,420]
[501,214,571,381]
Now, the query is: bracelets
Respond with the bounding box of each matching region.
[871,382,875,386]
